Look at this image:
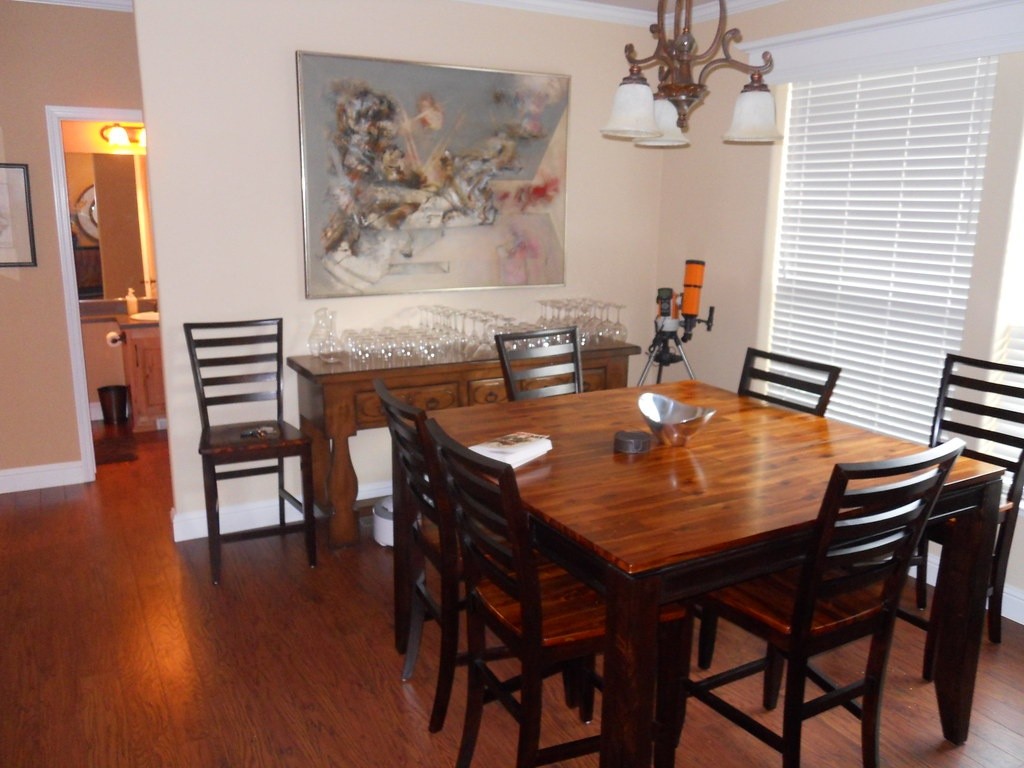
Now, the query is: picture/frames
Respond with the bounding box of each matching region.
[0,163,38,269]
[294,48,575,299]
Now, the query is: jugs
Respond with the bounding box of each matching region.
[309,307,344,364]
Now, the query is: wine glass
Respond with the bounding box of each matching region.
[416,303,517,361]
[537,298,628,346]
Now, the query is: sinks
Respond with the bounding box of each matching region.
[129,311,159,321]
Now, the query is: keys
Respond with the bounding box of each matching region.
[240,427,274,438]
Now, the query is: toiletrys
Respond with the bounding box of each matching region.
[125,288,138,314]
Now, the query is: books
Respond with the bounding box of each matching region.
[468,432,552,469]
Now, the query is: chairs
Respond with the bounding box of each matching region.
[495,326,584,399]
[370,379,515,731]
[697,346,839,668]
[691,438,965,767]
[428,414,687,768]
[831,355,1024,678]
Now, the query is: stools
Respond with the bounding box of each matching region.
[183,316,316,577]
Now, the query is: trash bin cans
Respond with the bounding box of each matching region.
[96,385,129,426]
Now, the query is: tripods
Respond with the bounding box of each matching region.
[637,318,695,386]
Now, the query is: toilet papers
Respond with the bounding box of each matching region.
[106,331,122,347]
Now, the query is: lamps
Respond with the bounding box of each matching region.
[604,1,782,147]
[101,120,146,145]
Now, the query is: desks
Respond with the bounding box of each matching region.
[406,382,1007,768]
[286,334,641,549]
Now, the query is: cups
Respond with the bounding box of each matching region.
[513,320,571,350]
[340,325,458,370]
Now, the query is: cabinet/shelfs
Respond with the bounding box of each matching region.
[117,322,167,434]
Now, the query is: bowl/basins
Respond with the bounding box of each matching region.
[637,392,717,448]
